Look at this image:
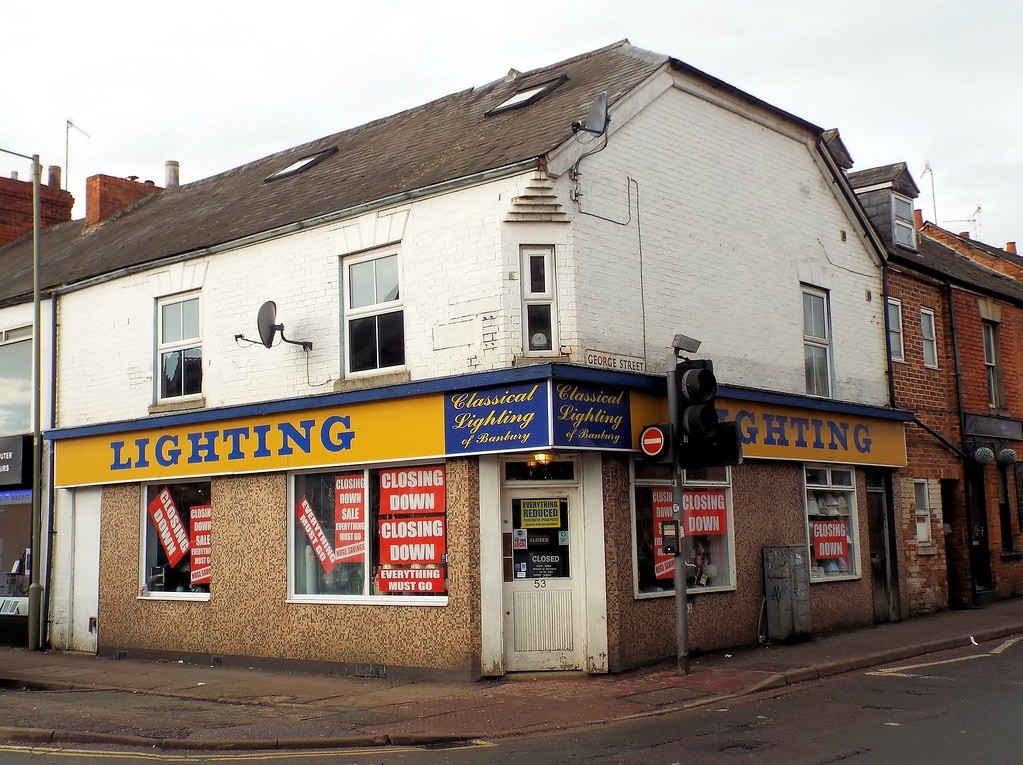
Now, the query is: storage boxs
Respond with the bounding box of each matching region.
[762,545,816,647]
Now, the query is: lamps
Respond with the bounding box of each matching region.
[991,438,1017,466]
[966,433,996,465]
[524,462,541,469]
[531,451,557,466]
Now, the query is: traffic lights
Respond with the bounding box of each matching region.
[674,359,725,467]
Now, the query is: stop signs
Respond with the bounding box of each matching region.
[641,427,665,455]
